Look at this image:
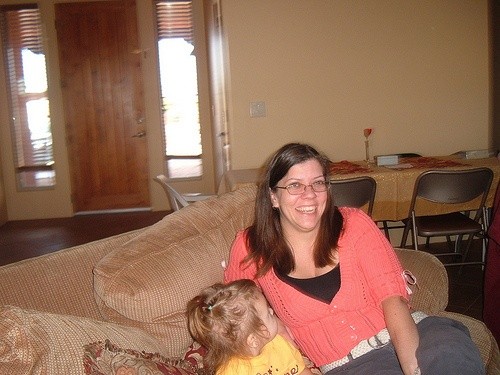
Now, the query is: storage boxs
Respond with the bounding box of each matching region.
[374,154,399,166]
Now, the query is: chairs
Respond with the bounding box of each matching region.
[153,174,214,212]
[330,151,493,274]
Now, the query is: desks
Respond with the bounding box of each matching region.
[218,155,500,251]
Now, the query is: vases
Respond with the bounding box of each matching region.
[366,141,370,163]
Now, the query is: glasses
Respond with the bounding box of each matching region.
[275,181,332,195]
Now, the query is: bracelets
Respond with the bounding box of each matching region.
[223,142,486,375]
[415,367,421,375]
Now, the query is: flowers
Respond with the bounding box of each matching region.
[363,128,372,138]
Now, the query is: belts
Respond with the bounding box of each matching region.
[320,311,427,375]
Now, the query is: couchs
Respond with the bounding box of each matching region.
[0,202,500,375]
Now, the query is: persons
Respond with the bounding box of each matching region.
[184,279,315,375]
